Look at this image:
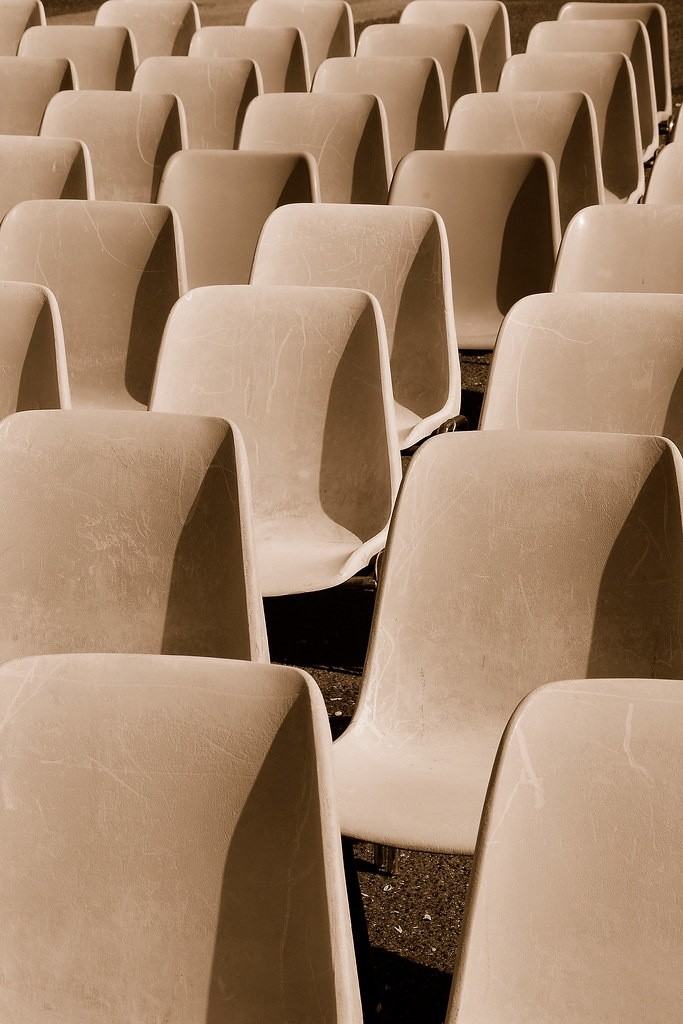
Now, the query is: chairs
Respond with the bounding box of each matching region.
[3,656,369,1024]
[322,430,682,859]
[389,149,563,352]
[145,284,397,599]
[551,202,681,295]
[245,203,461,454]
[475,288,683,426]
[0,1,682,224]
[444,676,682,1024]
[0,277,70,415]
[155,149,323,291]
[2,200,189,410]
[0,406,265,658]
